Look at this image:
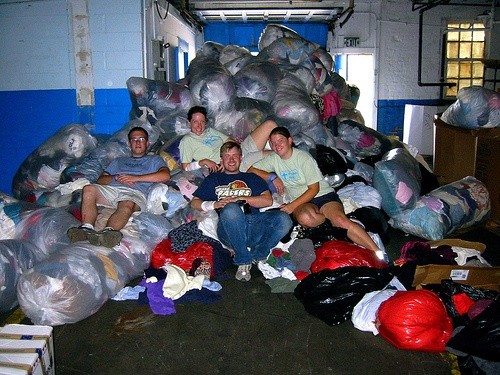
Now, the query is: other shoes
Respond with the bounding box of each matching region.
[252,258,266,265]
[235,264,253,282]
[376,251,389,263]
[323,173,345,188]
[67,226,96,242]
[87,229,123,248]
[349,217,365,229]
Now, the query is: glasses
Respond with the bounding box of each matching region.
[130,137,145,142]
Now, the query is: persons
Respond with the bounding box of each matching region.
[247,126,385,259]
[68,128,170,247]
[179,107,277,170]
[190,142,293,281]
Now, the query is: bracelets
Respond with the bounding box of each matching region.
[270,174,278,182]
[114,175,117,181]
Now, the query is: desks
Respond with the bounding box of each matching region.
[430,112,500,222]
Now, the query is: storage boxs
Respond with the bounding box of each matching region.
[0,323,54,374]
[403,238,499,291]
